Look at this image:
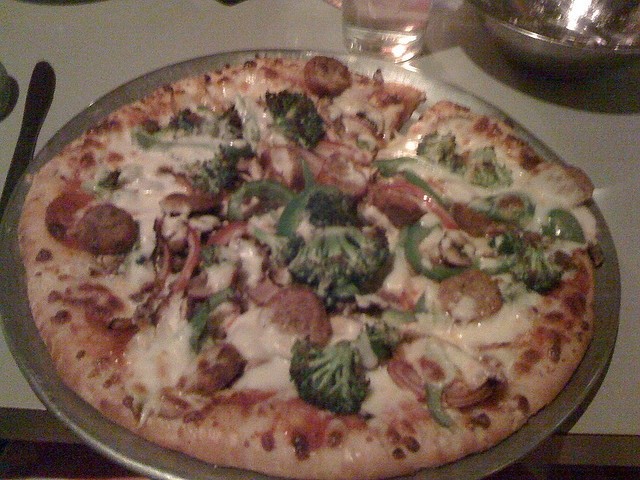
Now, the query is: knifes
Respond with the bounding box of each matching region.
[0,60,56,215]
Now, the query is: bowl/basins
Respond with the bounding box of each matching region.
[464,1,640,82]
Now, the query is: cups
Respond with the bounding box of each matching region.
[342,0,436,63]
